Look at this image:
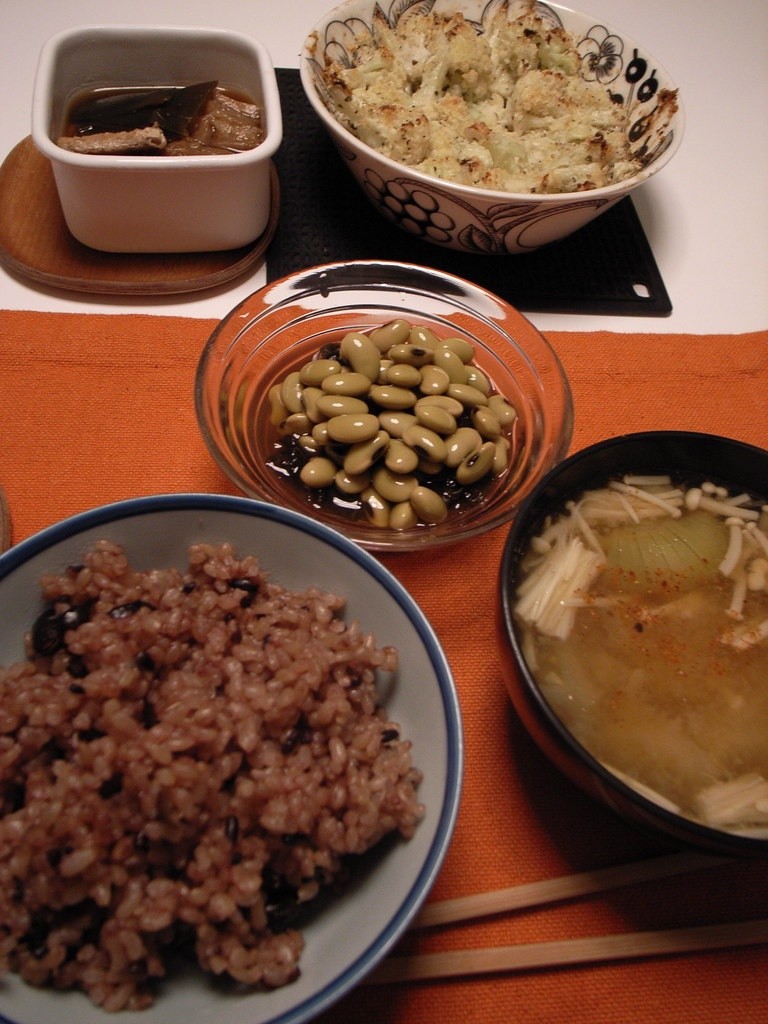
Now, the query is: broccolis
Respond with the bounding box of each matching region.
[327,7,635,195]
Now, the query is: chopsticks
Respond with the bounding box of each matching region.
[351,848,768,986]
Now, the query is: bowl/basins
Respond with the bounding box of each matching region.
[31,23,282,255]
[0,492,463,1024]
[493,429,768,849]
[194,257,573,557]
[300,0,685,263]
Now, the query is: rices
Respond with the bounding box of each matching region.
[0,538,428,1014]
